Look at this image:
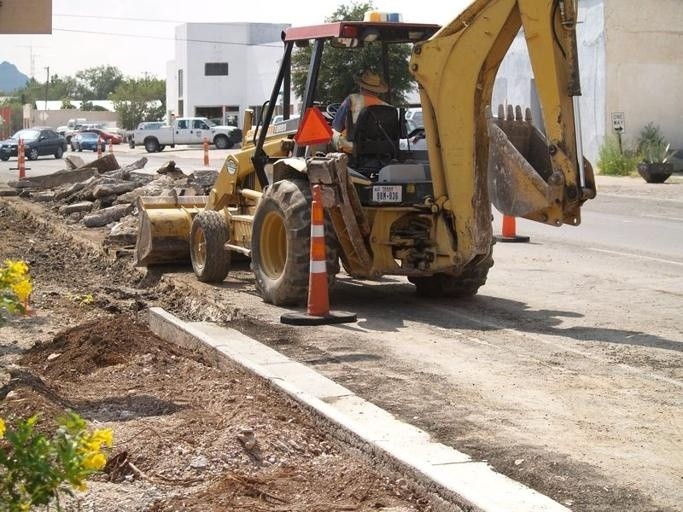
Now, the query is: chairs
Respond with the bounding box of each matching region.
[350,104,402,167]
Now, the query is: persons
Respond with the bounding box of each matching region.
[232,115,237,126]
[331,69,389,153]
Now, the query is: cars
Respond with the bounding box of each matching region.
[0,118,126,161]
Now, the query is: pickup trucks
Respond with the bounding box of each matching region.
[126,116,243,153]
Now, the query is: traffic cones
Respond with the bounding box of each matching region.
[491,214,530,243]
[97,135,103,159]
[9,138,31,181]
[203,135,209,167]
[108,139,113,153]
[280,184,357,326]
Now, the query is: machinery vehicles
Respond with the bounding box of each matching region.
[129,1,597,308]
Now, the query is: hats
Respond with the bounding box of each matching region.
[352,69,390,95]
